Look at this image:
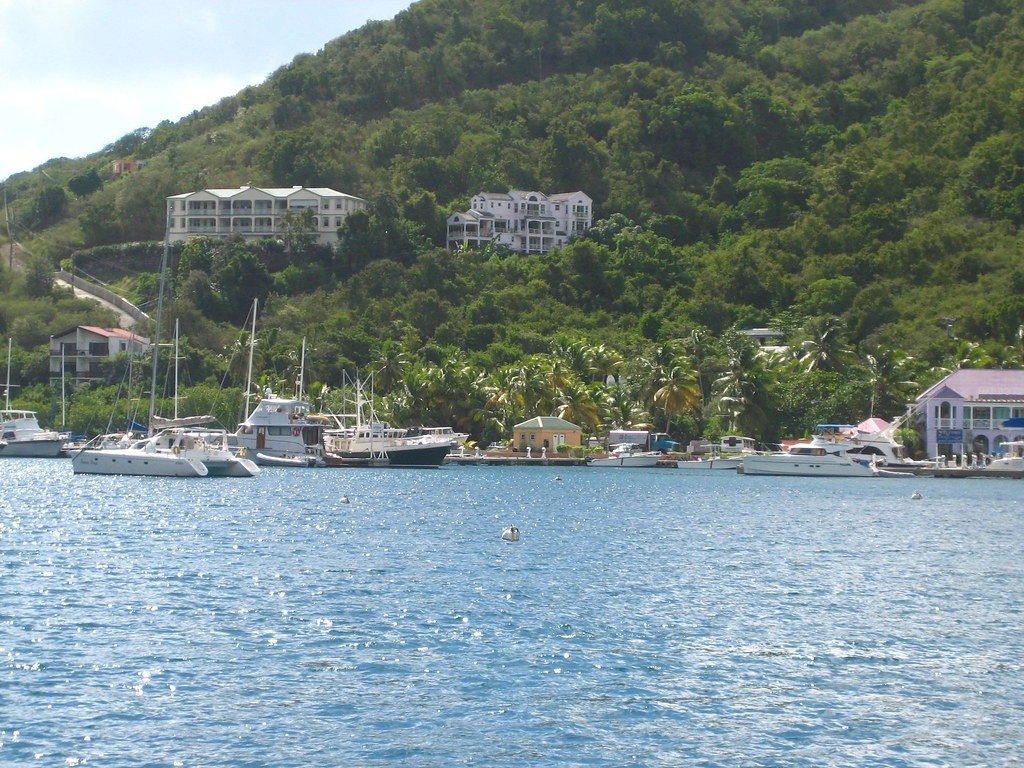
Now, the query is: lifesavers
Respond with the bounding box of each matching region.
[293,428,300,436]
[172,446,180,454]
[985,458,991,466]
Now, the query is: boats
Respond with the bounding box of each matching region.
[586,444,662,467]
[742,443,875,478]
[319,366,470,468]
[677,454,745,470]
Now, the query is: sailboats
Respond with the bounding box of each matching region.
[59,296,345,469]
[0,337,73,458]
[72,196,261,479]
